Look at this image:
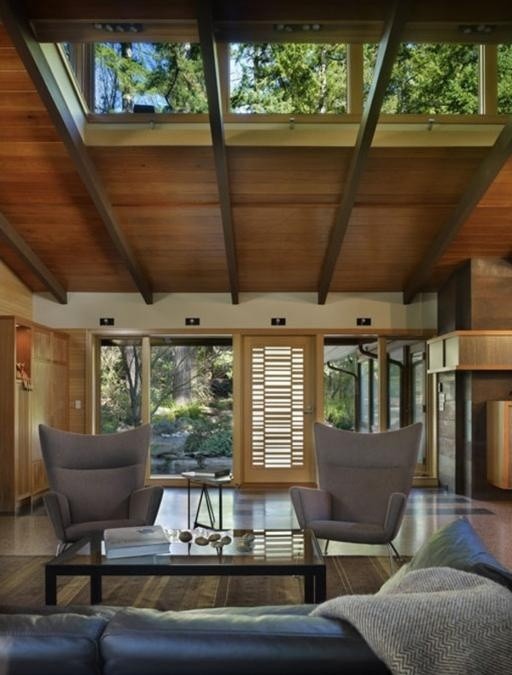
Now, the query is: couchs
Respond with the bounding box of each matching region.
[0,518,512,675]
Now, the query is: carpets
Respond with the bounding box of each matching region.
[1,555,413,612]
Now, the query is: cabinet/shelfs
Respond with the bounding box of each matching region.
[32,321,71,503]
[0,315,33,515]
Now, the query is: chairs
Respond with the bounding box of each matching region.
[38,424,163,558]
[289,423,423,579]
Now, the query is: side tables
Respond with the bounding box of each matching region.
[181,471,234,529]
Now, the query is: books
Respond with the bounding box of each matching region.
[104,525,172,558]
[194,467,230,478]
